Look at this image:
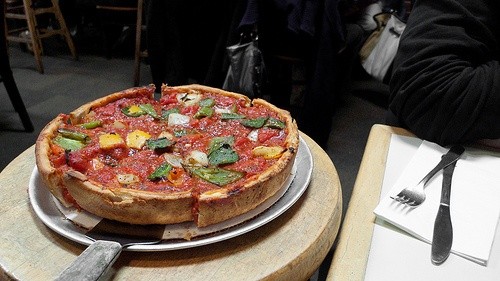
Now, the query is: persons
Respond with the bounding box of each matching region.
[386,0,500,148]
[234,0,365,110]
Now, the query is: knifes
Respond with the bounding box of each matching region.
[430,154,454,264]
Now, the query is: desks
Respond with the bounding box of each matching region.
[326,124,500,281]
[0,129,343,281]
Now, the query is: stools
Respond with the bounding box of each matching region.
[4,0,76,75]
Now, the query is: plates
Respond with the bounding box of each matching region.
[28,134,313,252]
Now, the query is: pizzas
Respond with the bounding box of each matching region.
[35,84,299,225]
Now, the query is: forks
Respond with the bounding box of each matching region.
[390,143,466,208]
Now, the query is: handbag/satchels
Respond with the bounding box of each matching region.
[362,15,407,84]
[222,42,265,98]
[359,13,391,58]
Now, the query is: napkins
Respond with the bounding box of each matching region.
[372,140,500,266]
